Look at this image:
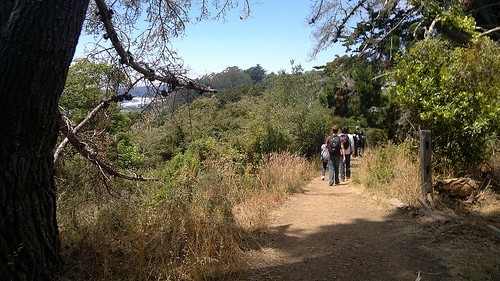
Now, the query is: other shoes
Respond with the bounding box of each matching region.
[329,181,333,186]
[346,176,350,179]
[335,181,340,184]
[321,176,325,180]
[341,177,345,182]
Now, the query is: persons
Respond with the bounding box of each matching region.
[320,137,329,180]
[326,126,345,186]
[338,126,354,182]
[353,126,366,158]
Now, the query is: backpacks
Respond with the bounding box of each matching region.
[328,135,341,160]
[343,135,349,150]
